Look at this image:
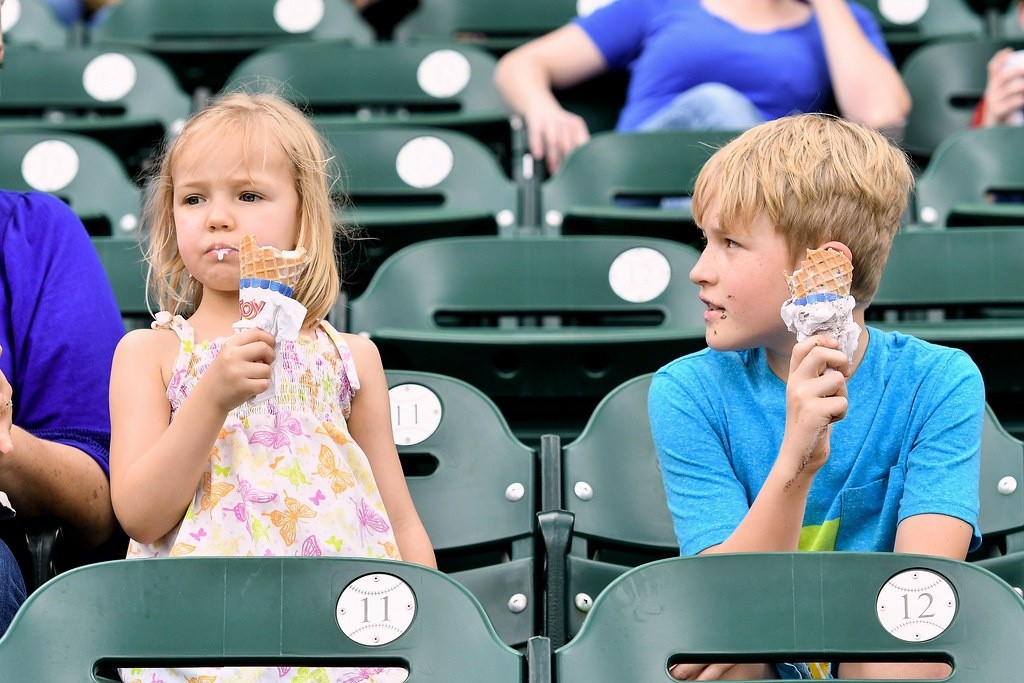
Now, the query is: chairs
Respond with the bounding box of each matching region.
[0,0,1024,683]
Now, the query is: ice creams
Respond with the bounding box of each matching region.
[233,235,307,364]
[781,248,861,377]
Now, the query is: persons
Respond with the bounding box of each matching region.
[0,186,127,640]
[971,0,1024,204]
[109,93,438,683]
[492,1,911,211]
[647,113,985,683]
